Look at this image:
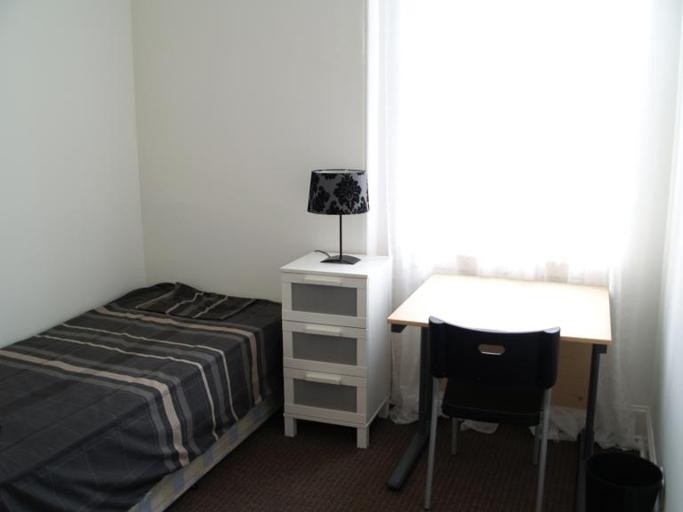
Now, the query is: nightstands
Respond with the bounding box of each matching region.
[278,253,394,451]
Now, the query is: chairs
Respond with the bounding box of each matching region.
[420,314,562,511]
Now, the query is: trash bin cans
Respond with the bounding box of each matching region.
[585,451,663,512]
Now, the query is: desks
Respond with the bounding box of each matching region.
[384,273,614,510]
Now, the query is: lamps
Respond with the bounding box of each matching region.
[305,167,370,266]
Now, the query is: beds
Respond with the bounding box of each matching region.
[0,280,281,511]
